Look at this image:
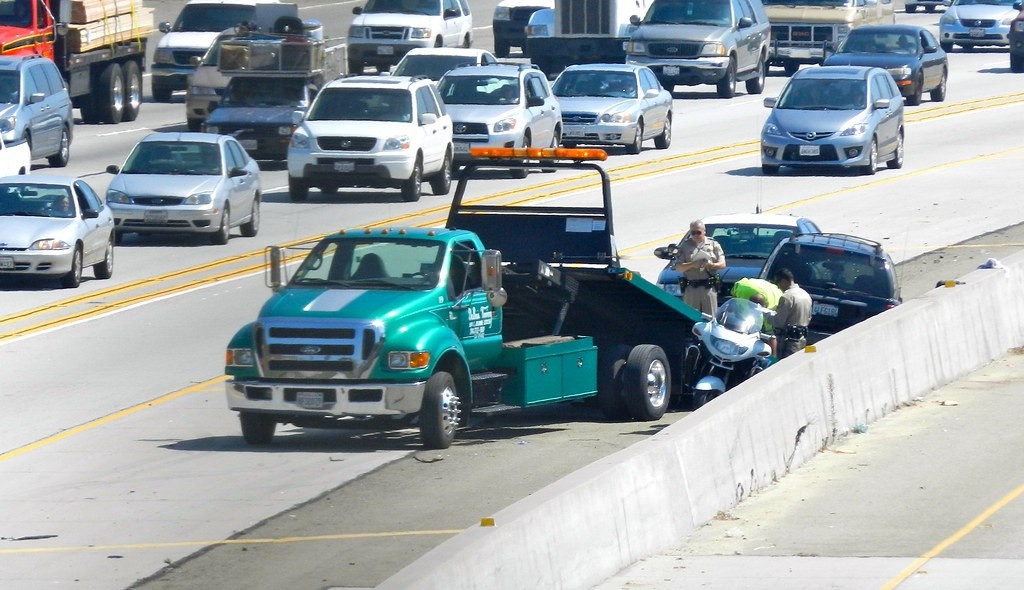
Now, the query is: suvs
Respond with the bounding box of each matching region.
[284,74,454,202]
[625,0,772,99]
[754,231,905,348]
[0,55,74,166]
[150,1,300,101]
[435,61,564,179]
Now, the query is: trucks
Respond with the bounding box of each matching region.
[0,0,148,123]
[224,146,779,447]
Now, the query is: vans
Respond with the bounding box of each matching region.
[186,28,327,133]
[760,0,897,76]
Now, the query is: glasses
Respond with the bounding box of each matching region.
[691,231,704,235]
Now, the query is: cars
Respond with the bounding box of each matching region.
[346,0,475,76]
[380,46,509,103]
[492,1,555,60]
[825,24,950,105]
[905,0,952,13]
[939,0,1023,54]
[550,65,674,155]
[0,175,113,288]
[100,132,265,244]
[197,70,327,164]
[652,213,827,311]
[760,66,907,178]
[1008,2,1024,72]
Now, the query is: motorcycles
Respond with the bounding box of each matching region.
[677,297,780,412]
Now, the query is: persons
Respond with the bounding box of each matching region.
[729,268,812,360]
[53,195,72,216]
[501,84,516,103]
[676,219,726,316]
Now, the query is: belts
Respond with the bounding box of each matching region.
[686,281,706,286]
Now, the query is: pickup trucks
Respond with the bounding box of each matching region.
[0,129,33,179]
